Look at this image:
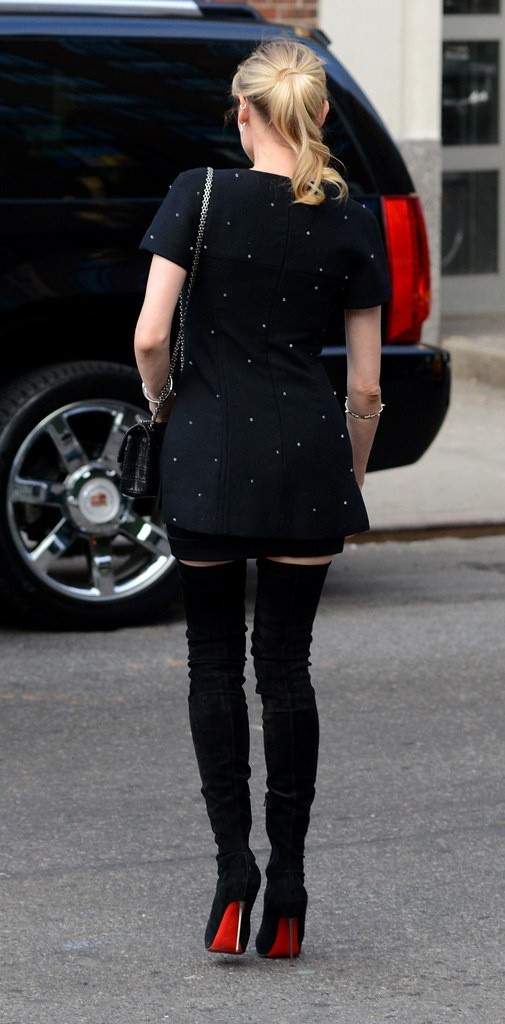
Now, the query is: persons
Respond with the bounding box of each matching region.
[115,35,386,957]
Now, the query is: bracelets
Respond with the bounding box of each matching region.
[142,376,175,403]
[345,396,385,419]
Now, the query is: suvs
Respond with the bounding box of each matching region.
[0,2,456,634]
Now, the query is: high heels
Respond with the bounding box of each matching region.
[255,872,309,967]
[205,853,262,956]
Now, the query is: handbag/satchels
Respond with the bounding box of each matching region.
[117,166,217,515]
